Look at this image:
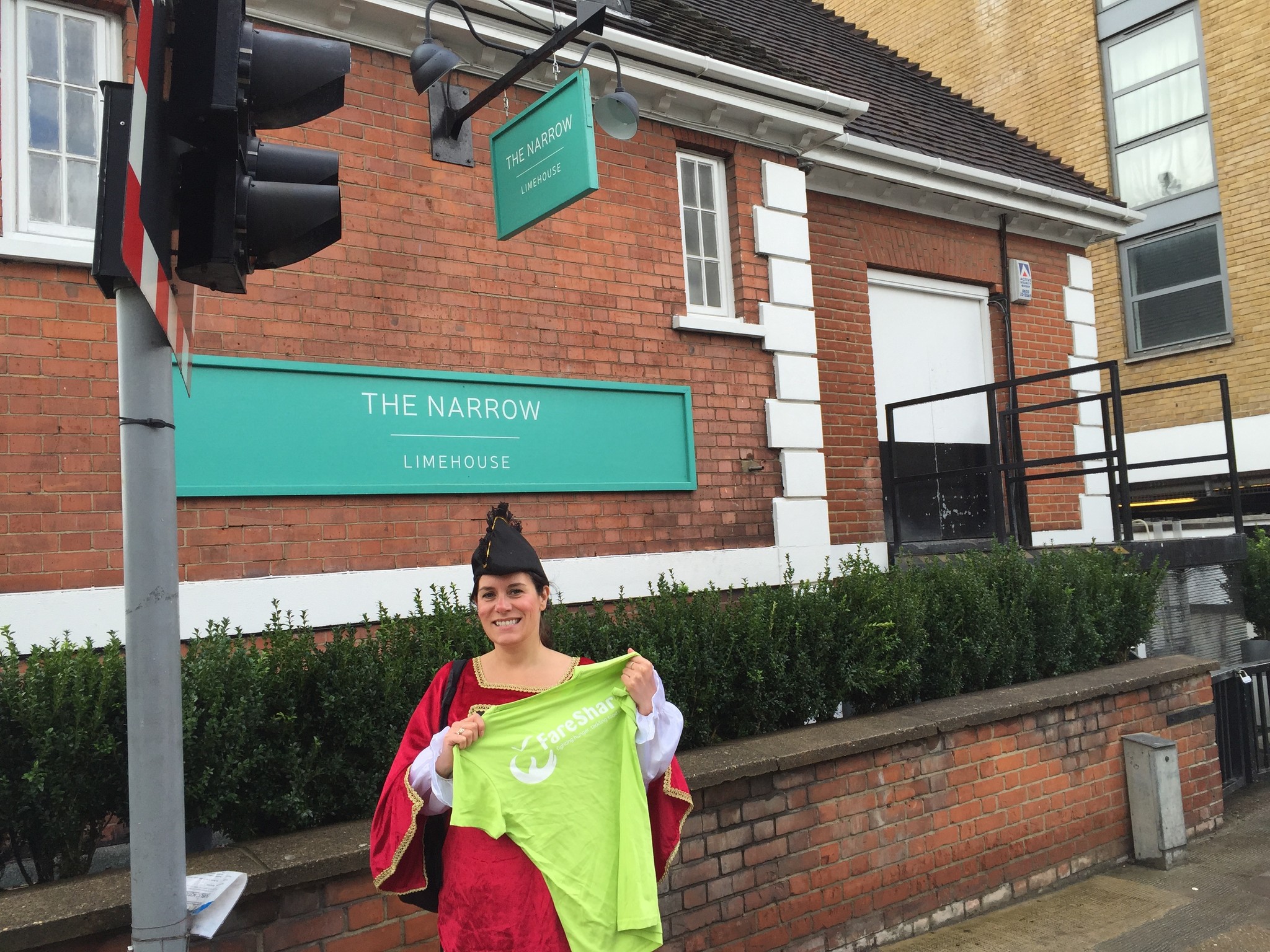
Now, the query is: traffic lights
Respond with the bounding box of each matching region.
[182,0,352,295]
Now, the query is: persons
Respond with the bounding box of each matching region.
[363,504,693,950]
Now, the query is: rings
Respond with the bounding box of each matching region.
[630,661,635,669]
[456,727,465,736]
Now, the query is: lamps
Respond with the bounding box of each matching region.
[409,0,641,168]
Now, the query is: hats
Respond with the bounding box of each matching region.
[469,500,548,580]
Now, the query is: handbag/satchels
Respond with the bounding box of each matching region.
[392,657,476,910]
